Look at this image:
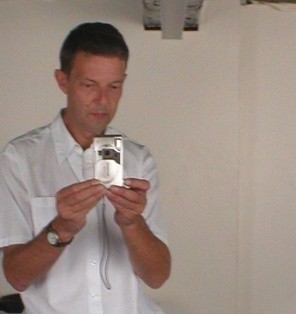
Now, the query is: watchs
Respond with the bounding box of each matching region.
[45,223,72,247]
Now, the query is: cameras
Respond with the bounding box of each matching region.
[92,134,125,189]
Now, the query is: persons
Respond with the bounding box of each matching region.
[0,21,171,314]
